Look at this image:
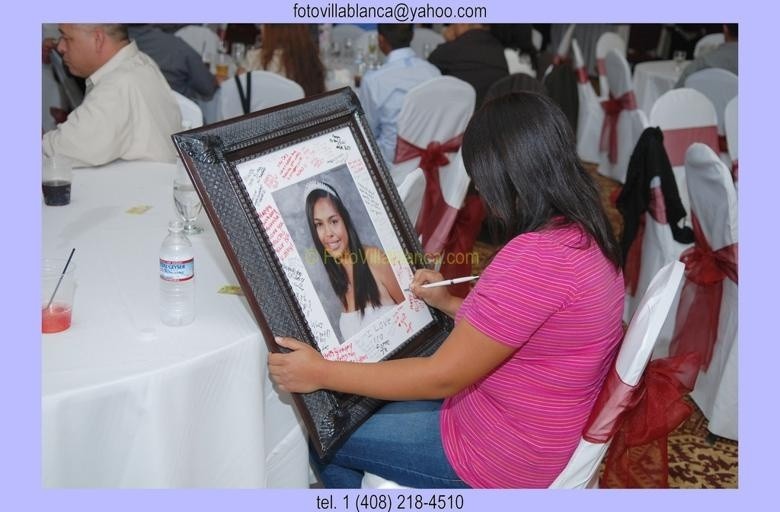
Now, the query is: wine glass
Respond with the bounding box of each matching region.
[172,154,204,235]
[674,44,711,74]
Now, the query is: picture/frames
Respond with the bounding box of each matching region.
[166,86,456,465]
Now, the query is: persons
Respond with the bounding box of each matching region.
[268,91,626,488]
[42,22,739,167]
[302,180,406,341]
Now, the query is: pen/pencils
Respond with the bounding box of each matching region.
[403,275,480,292]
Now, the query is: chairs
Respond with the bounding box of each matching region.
[391,72,486,282]
[168,27,448,130]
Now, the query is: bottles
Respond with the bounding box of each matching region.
[201,34,431,89]
[158,219,196,327]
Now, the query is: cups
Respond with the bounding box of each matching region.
[40,259,81,333]
[41,156,77,207]
